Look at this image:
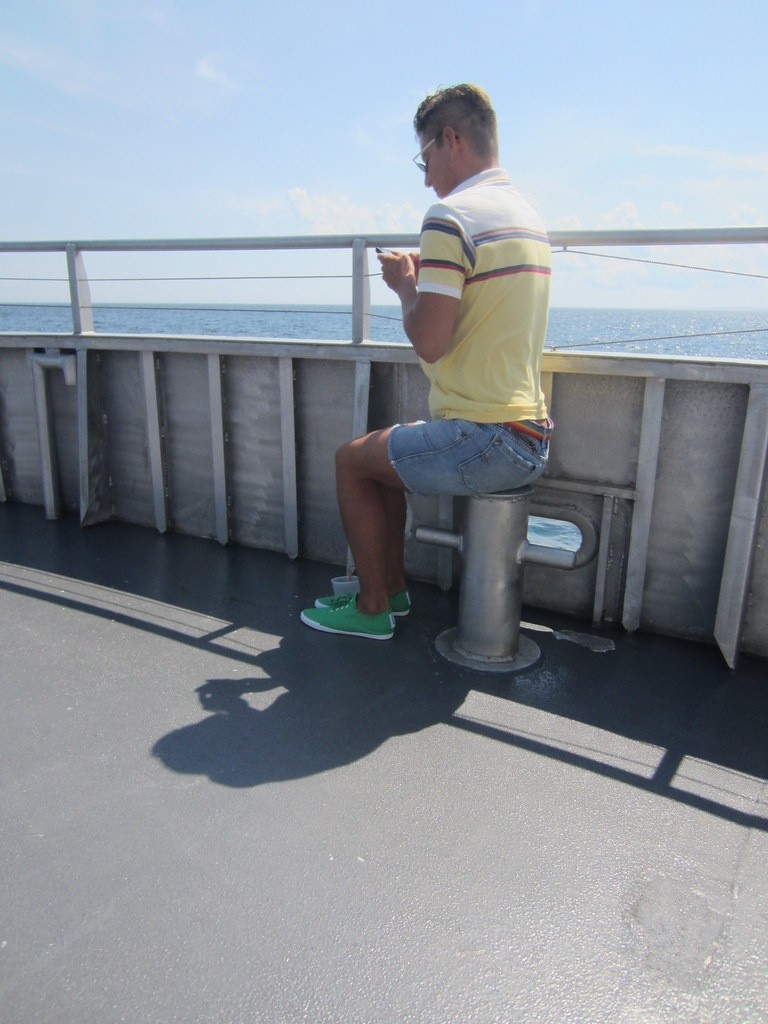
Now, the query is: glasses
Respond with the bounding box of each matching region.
[412,129,459,173]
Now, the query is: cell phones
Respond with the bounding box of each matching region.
[376,247,397,257]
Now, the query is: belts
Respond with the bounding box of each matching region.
[504,416,554,441]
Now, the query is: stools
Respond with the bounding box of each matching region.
[415,488,541,672]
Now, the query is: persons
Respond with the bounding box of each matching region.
[296,82,558,644]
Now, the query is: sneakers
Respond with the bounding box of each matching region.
[314,585,413,617]
[299,593,396,640]
